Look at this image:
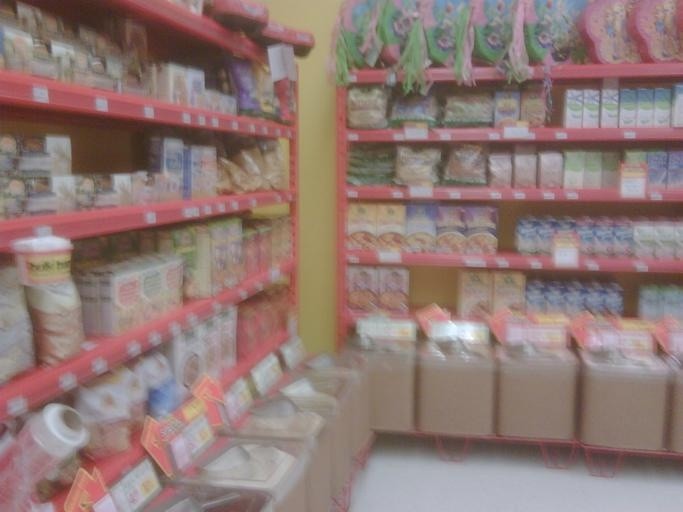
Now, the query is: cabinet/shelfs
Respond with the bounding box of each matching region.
[335,61,683,477]
[1,0,315,512]
